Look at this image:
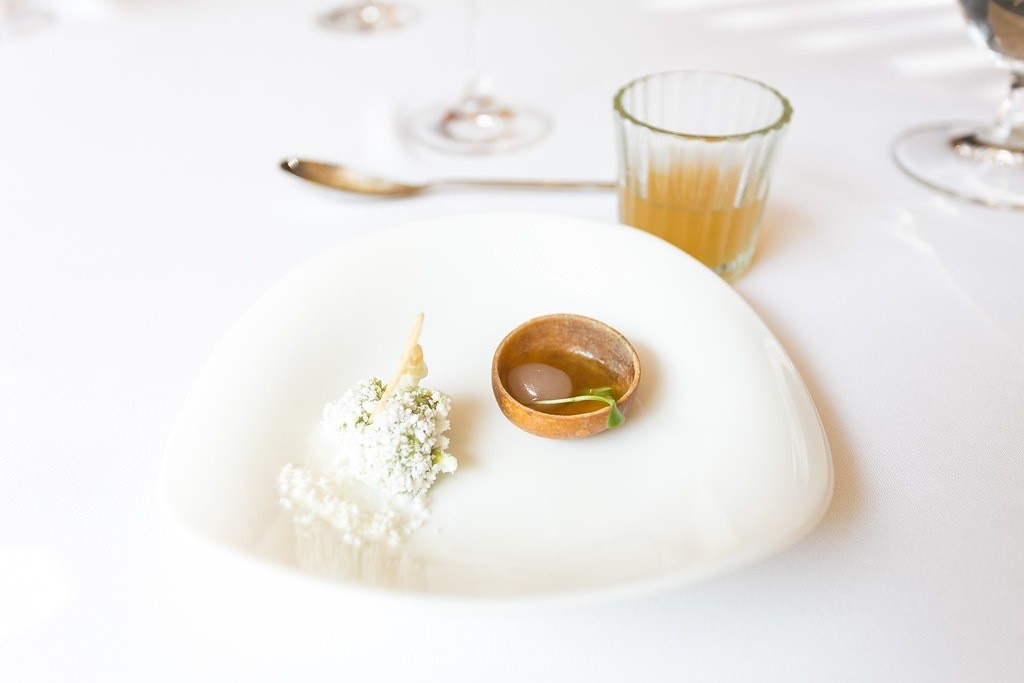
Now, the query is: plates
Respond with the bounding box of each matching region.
[183,184,834,609]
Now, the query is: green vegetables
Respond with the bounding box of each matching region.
[521,386,625,428]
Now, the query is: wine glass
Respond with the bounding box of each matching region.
[887,0,1024,212]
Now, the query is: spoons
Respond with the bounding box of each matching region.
[279,157,618,200]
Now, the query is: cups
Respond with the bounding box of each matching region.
[609,64,792,282]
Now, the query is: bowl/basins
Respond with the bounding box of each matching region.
[491,314,641,438]
